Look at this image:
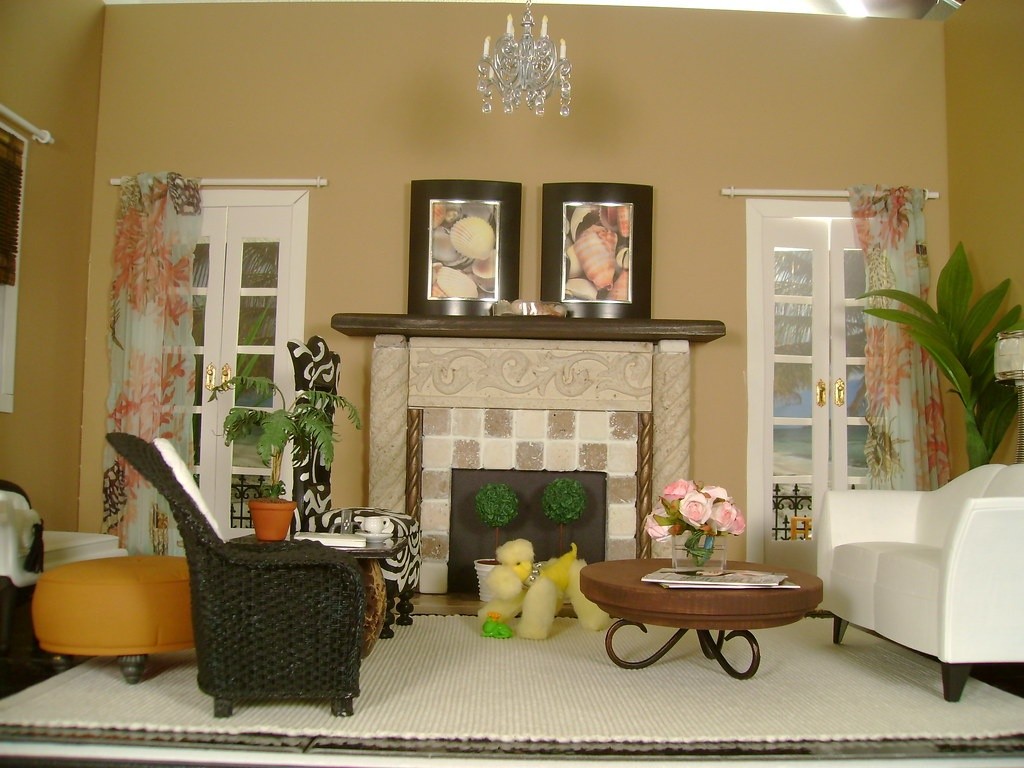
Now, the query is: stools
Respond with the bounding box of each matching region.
[31,555,196,686]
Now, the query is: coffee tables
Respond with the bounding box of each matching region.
[580,558,823,680]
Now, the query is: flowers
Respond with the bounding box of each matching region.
[644,478,746,544]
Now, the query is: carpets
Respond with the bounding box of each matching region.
[1,613,1024,745]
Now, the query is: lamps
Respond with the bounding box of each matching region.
[993,330,1024,465]
[476,0,572,116]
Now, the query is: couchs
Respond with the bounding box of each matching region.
[817,465,1024,703]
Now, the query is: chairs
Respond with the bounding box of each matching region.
[0,480,52,660]
[152,438,227,545]
[105,431,366,719]
[286,335,421,639]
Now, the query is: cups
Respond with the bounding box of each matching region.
[341,510,353,534]
[364,517,389,534]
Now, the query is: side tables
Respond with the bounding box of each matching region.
[230,533,408,661]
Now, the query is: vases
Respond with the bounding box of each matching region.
[672,531,727,571]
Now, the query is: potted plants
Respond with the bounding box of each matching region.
[208,374,361,541]
[540,477,588,604]
[473,483,519,603]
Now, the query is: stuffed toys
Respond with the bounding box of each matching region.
[475,538,610,639]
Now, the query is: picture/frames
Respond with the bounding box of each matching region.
[407,179,523,317]
[539,182,653,320]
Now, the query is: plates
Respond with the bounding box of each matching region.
[355,532,394,542]
